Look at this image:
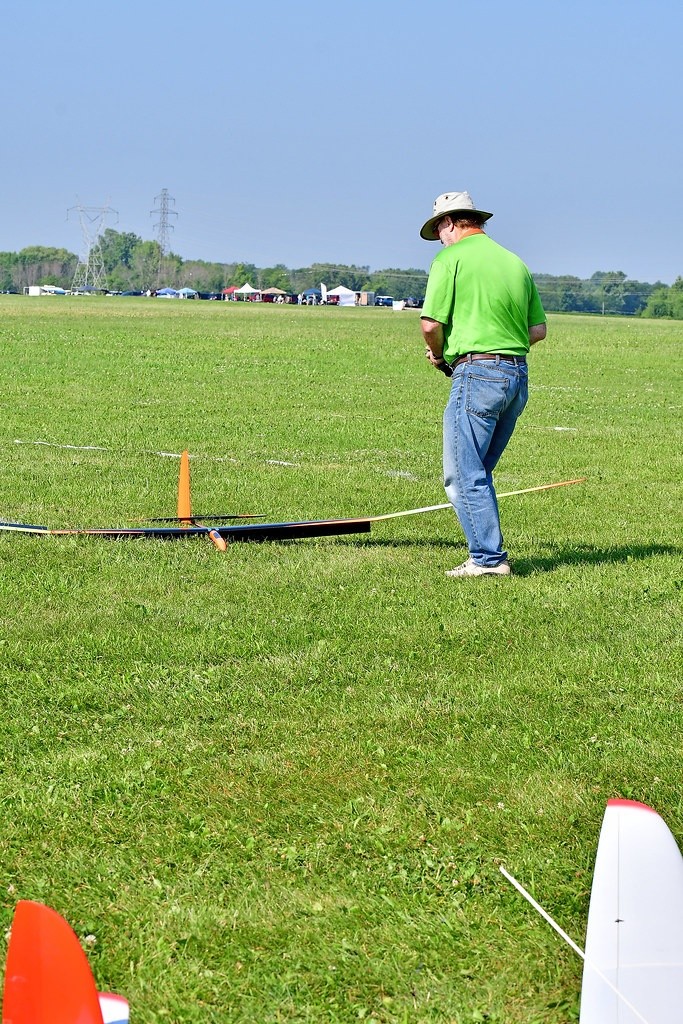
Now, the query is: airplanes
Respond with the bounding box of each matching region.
[1,449,593,551]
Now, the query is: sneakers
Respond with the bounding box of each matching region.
[444,557,514,578]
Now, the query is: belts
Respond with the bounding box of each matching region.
[451,353,525,371]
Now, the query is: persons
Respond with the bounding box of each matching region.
[277,295,284,302]
[419,191,548,577]
[297,294,302,305]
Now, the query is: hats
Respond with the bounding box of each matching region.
[420,192,493,241]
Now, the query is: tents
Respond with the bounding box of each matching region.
[156,283,357,307]
[77,285,97,295]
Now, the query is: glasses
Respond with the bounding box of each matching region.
[432,214,452,238]
